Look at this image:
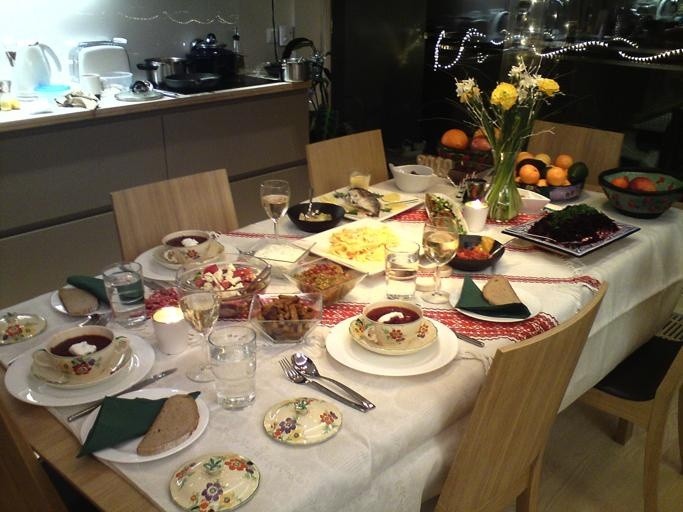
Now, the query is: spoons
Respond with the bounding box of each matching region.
[290,352,375,413]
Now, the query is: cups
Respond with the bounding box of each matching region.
[381,239,420,302]
[100,261,149,329]
[0,80,11,93]
[79,72,100,95]
[159,229,224,267]
[391,161,435,195]
[350,300,438,356]
[206,324,258,414]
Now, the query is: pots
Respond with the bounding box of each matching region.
[264,58,318,86]
[137,33,245,88]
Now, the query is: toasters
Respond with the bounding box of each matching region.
[66,40,132,86]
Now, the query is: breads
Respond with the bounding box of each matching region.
[58,287,100,315]
[482,275,523,306]
[137,394,199,456]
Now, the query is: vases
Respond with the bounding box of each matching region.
[484,148,524,224]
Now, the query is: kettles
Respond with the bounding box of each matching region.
[5,40,64,102]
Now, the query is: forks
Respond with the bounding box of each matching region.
[277,355,367,415]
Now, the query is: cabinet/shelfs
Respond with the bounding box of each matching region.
[1,90,310,310]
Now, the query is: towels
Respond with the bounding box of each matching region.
[68,269,146,305]
[455,273,531,318]
[72,391,203,458]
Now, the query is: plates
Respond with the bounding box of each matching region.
[0,313,45,344]
[132,244,242,283]
[293,217,417,275]
[315,182,422,223]
[327,305,459,376]
[449,283,544,324]
[263,397,341,446]
[4,330,154,407]
[498,209,641,257]
[166,449,261,509]
[49,274,156,315]
[81,389,210,462]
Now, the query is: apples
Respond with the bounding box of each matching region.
[470,136,492,152]
[609,177,629,190]
[472,127,500,144]
[630,177,656,192]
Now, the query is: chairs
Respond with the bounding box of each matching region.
[529,121,627,191]
[424,281,615,509]
[109,169,238,264]
[304,128,388,197]
[578,336,683,509]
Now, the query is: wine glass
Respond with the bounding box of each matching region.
[178,281,221,385]
[419,216,459,303]
[256,179,292,241]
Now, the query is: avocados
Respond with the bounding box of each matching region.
[567,162,588,184]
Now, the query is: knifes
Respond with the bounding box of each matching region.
[66,368,178,422]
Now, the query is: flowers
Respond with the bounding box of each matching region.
[451,54,565,216]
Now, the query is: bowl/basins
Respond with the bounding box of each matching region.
[598,166,680,219]
[245,290,322,345]
[244,237,317,275]
[175,253,272,317]
[447,233,506,271]
[100,69,133,93]
[286,202,347,234]
[282,255,369,304]
